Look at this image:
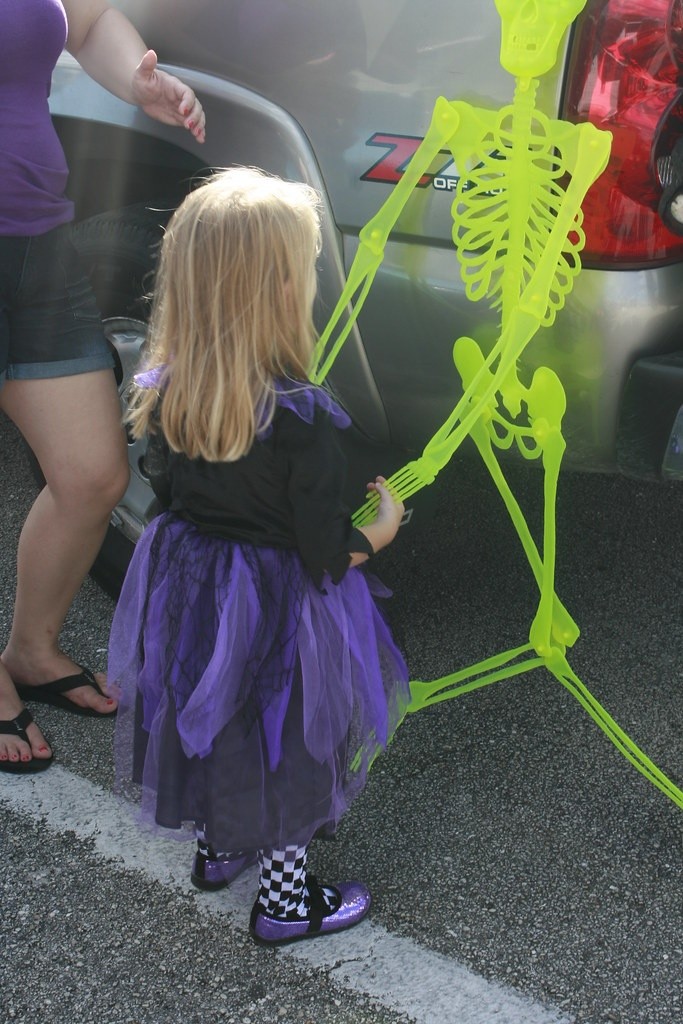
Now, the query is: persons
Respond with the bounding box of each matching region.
[107,165,407,945]
[0,2,205,773]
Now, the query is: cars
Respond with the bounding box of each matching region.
[40,0,683,618]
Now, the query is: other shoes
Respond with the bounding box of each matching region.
[248,877,372,946]
[190,848,258,891]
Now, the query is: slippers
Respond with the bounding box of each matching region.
[0,709,53,774]
[12,661,118,717]
